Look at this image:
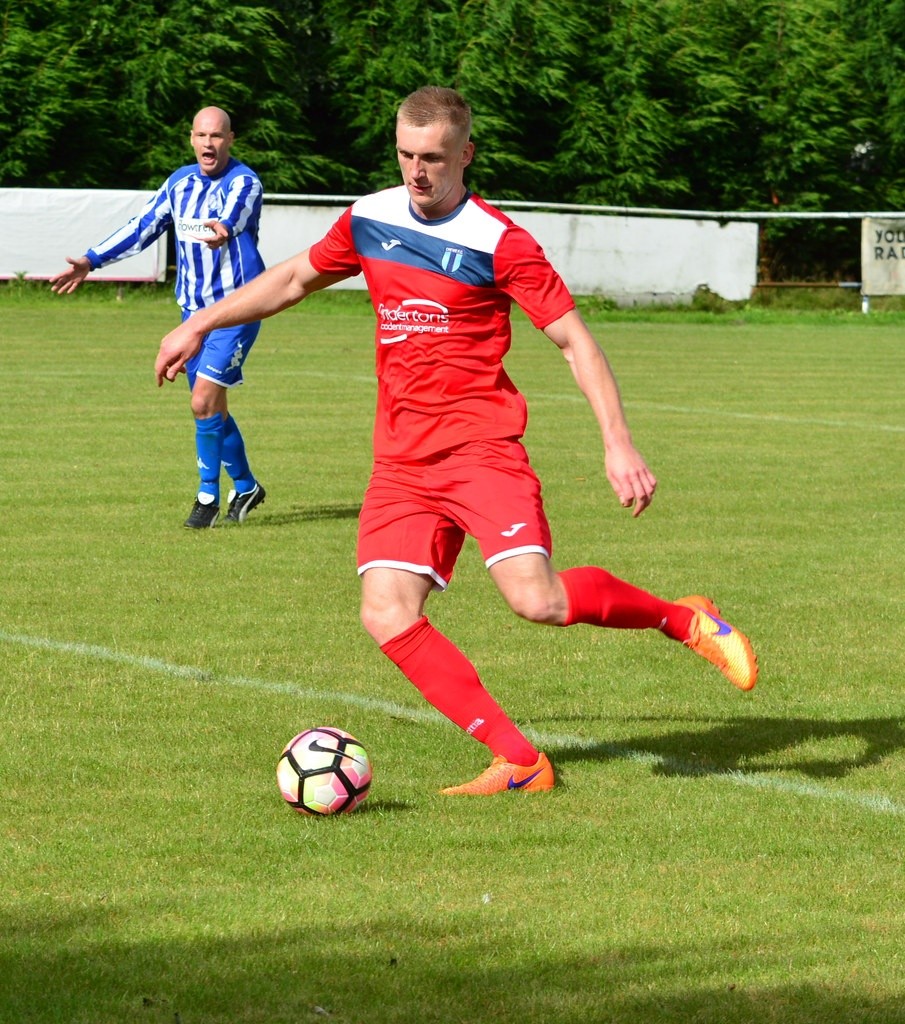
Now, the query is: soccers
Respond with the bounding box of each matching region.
[276,726,373,815]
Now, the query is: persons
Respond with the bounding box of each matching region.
[48,106,268,531]
[154,87,760,796]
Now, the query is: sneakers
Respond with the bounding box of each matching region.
[676,594,759,692]
[440,750,554,795]
[183,491,221,528]
[228,480,267,521]
